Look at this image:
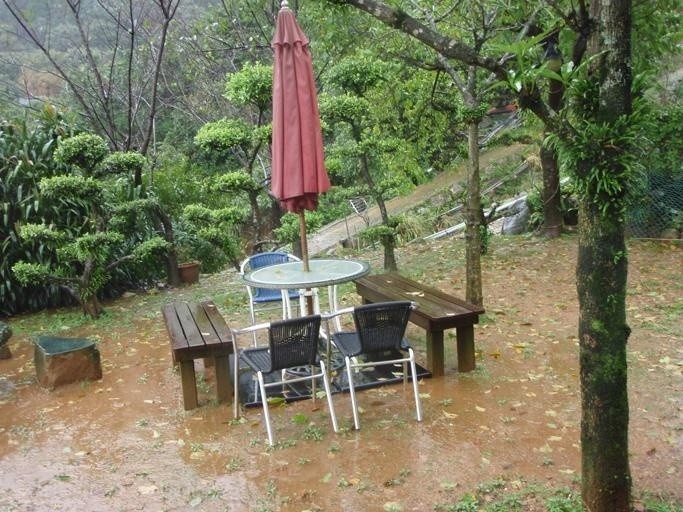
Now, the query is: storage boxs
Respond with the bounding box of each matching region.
[161,300,235,411]
[351,271,484,377]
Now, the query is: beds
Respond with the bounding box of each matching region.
[267,1,330,316]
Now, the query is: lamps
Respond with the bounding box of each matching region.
[230,250,424,447]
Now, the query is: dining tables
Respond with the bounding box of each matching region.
[174,246,203,283]
